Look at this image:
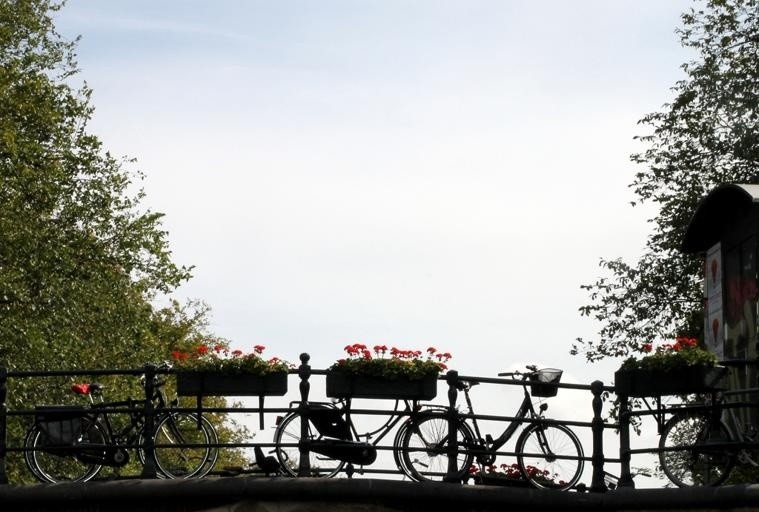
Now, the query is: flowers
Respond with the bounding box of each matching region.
[620,337,715,374]
[171,345,295,376]
[468,464,567,485]
[325,344,452,382]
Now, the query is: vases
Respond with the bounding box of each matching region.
[326,374,437,401]
[178,372,287,396]
[615,364,705,397]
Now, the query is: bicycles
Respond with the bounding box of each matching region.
[273,398,478,483]
[24,360,219,485]
[393,363,585,493]
[660,360,757,490]
